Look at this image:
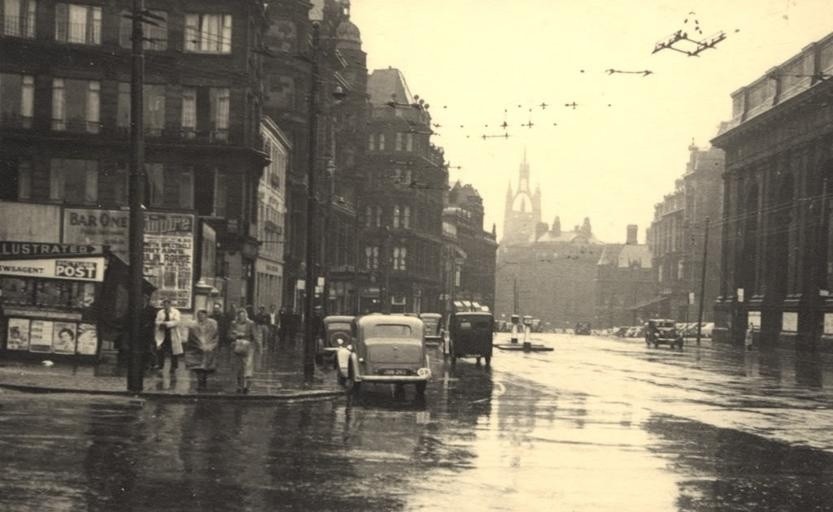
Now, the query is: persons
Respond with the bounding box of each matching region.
[744,322,754,351]
[145,298,288,394]
[58,328,74,351]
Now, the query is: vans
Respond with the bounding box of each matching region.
[575,320,591,336]
[437,312,494,365]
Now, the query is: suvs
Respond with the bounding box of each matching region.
[314,315,359,366]
[646,318,684,350]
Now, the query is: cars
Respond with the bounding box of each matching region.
[495,317,543,332]
[335,312,431,393]
[613,322,715,338]
[419,312,444,347]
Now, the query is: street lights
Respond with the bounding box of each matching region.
[238,235,262,317]
[305,77,348,386]
